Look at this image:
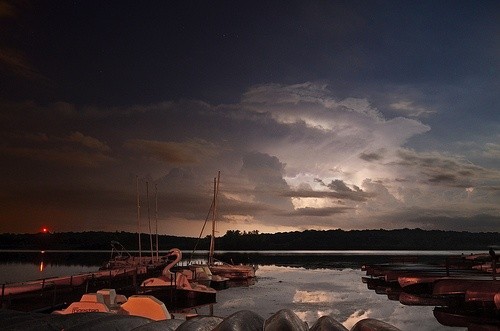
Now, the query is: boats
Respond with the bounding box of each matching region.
[362,259,500,310]
[101,242,256,308]
[2,283,409,331]
